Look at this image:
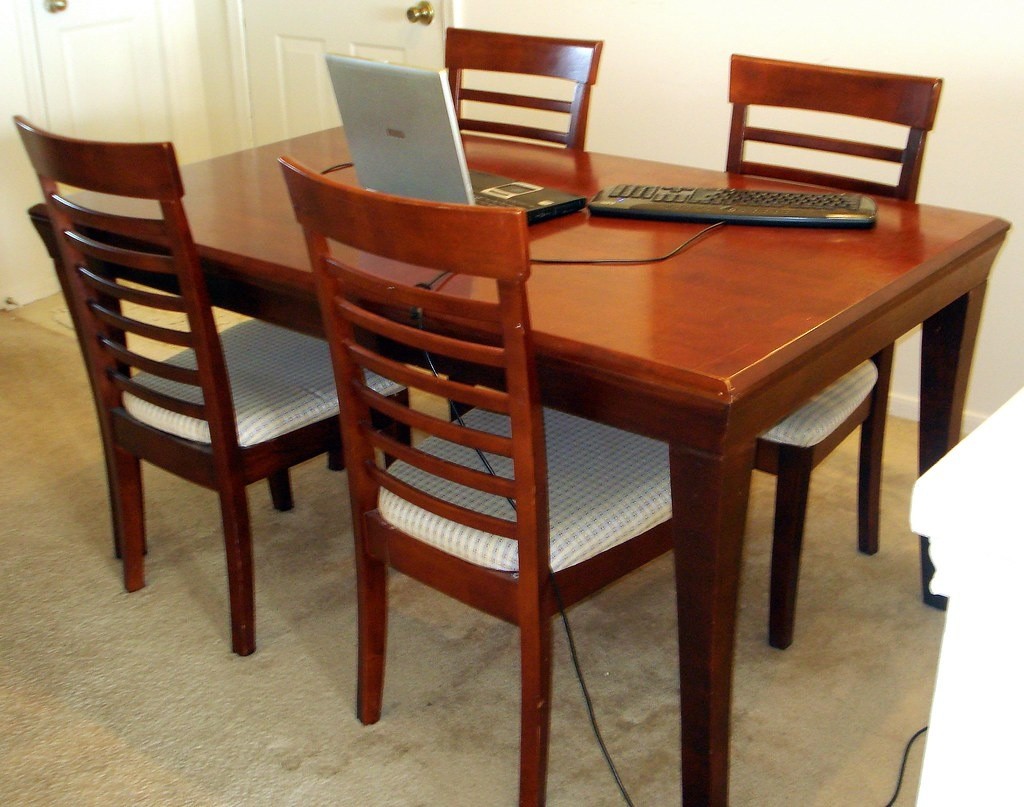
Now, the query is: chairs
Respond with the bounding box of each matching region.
[328,26,603,469]
[278,158,672,807]
[726,53,942,651]
[15,114,411,654]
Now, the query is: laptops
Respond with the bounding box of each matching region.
[323,52,586,231]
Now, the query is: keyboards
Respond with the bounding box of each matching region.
[589,184,878,226]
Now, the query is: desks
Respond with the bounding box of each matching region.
[28,123,1011,807]
